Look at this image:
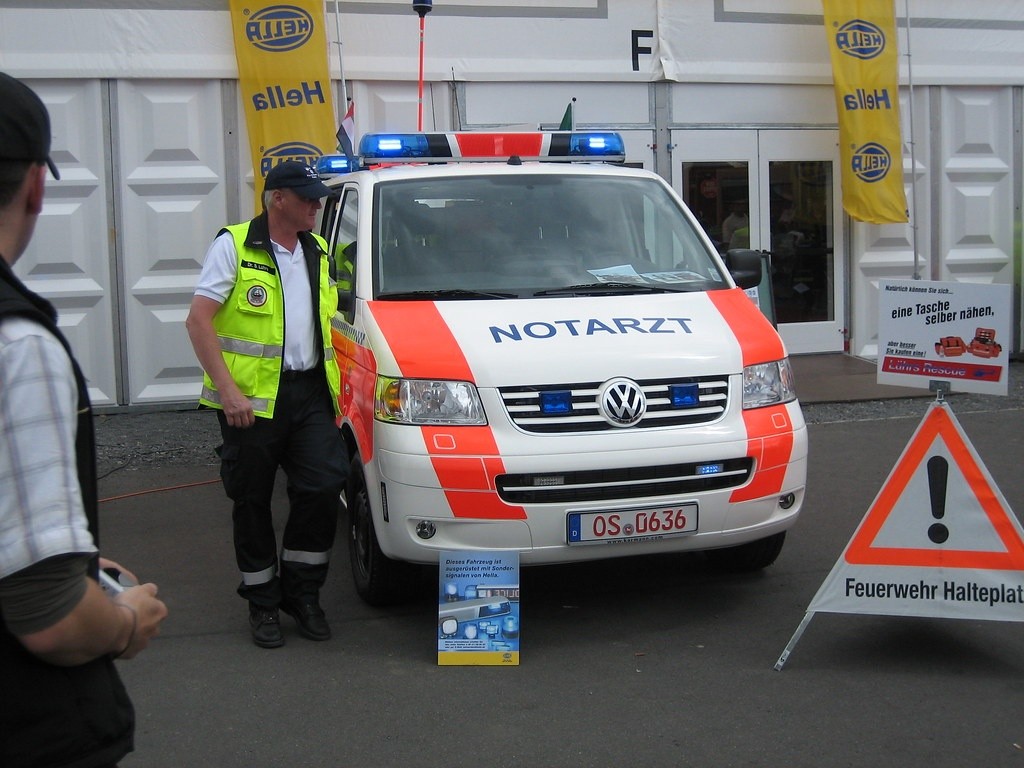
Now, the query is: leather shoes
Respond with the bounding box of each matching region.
[280,593,330,641]
[248,605,283,648]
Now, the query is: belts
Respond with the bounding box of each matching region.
[281,367,319,381]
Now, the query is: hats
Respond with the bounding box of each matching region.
[263,161,336,199]
[0,70,61,180]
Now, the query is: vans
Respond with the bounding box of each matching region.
[307,131,810,611]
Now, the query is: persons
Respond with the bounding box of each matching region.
[721,200,750,251]
[186,160,351,648]
[0,71,168,768]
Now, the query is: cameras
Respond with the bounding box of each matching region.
[98,566,135,600]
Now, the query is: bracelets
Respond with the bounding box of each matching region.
[111,601,137,657]
